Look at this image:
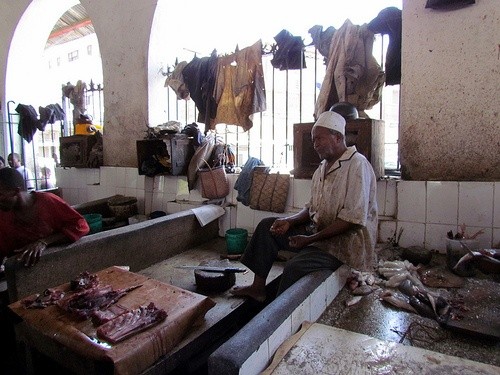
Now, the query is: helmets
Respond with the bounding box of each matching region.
[329,102,357,120]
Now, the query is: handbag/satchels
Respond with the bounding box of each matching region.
[196,154,229,199]
[250,167,289,213]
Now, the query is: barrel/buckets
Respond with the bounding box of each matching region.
[107,195,137,220]
[445,234,479,272]
[225,228,248,254]
[83,214,103,233]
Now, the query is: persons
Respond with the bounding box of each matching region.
[0,167,90,267]
[8,153,33,188]
[230,111,377,302]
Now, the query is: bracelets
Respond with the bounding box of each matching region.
[39,240,48,246]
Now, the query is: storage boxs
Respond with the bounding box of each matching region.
[136,139,196,176]
[60,135,97,167]
[294,120,385,180]
[445,237,481,268]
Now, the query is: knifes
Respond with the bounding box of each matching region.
[173,265,247,273]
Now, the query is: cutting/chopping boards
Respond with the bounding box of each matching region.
[193,256,236,293]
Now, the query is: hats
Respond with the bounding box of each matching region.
[312,111,347,135]
